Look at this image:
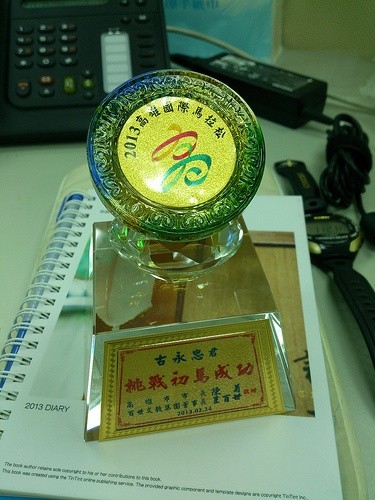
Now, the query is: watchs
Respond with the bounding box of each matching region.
[273,159,375,367]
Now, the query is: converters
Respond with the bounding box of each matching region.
[191,52,328,128]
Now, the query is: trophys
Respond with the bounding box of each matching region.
[84,70,296,442]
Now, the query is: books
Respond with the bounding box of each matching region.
[1,187,341,500]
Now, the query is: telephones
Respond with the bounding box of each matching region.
[1,0,173,148]
